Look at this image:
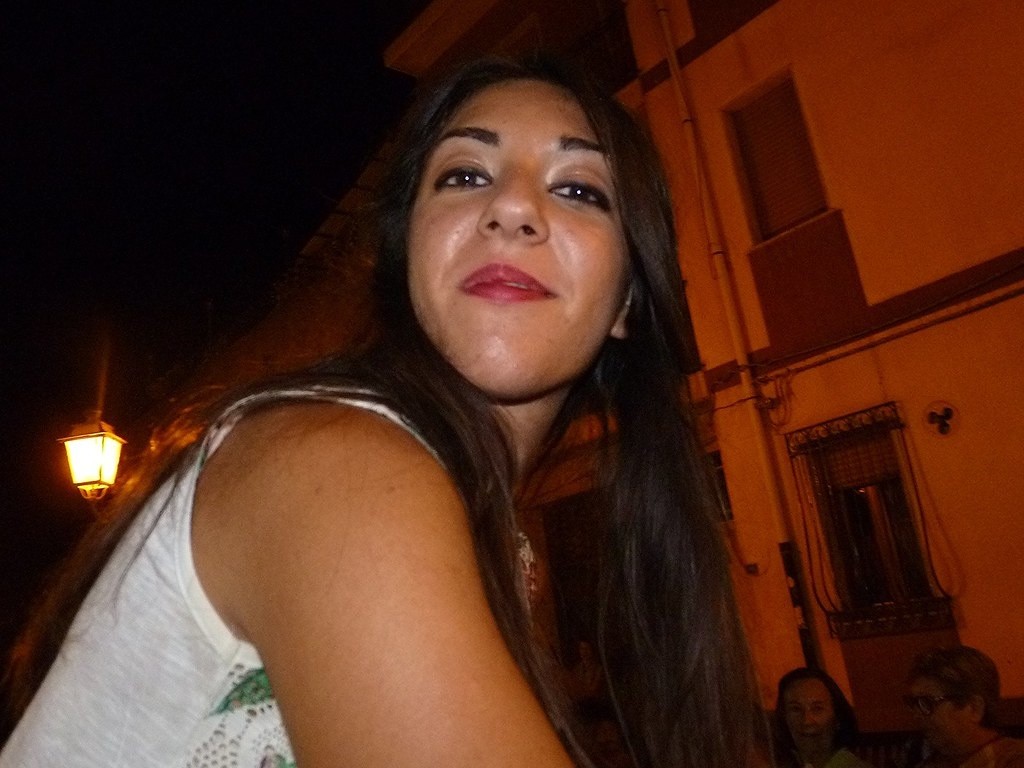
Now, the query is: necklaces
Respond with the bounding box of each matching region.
[493,465,536,630]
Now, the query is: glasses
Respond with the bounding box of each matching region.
[900,694,954,716]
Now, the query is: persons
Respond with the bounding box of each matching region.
[0,56,779,768]
[892,641,1024,767]
[772,663,869,768]
